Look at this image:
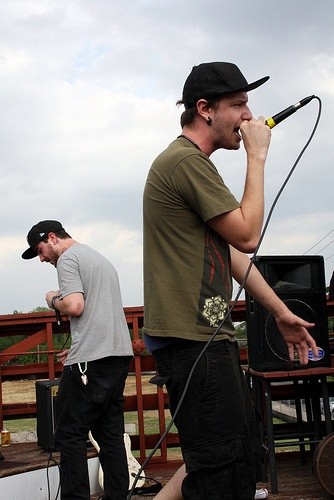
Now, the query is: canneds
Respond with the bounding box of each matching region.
[1,429,10,447]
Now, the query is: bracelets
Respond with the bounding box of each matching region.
[51,296,60,308]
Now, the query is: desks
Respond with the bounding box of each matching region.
[241,364,334,496]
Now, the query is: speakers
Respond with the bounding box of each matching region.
[36,379,95,451]
[244,255,331,372]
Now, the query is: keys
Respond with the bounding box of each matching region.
[81,375,87,385]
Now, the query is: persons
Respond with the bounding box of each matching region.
[142,62,319,500]
[21,220,134,500]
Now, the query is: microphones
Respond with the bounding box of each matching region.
[265,95,316,128]
[54,309,62,330]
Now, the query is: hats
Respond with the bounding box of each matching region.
[20,220,62,260]
[175,62,270,107]
